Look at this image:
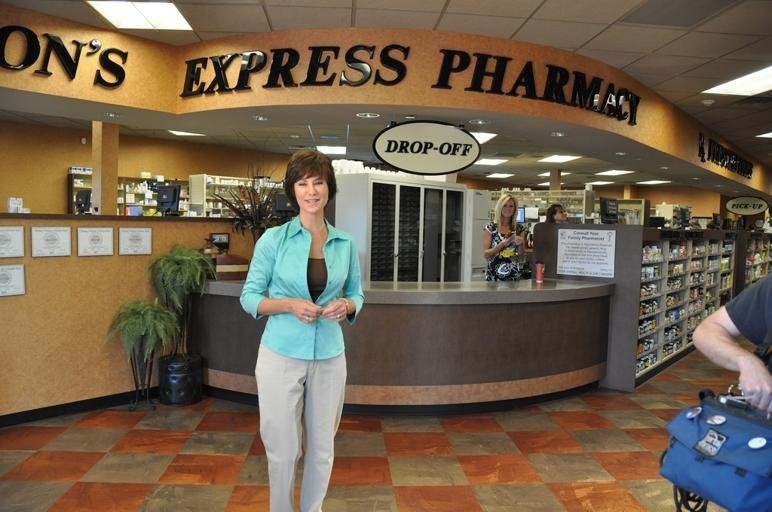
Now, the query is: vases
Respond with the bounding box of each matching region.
[249,226,263,244]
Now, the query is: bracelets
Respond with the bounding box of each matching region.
[304,316,309,321]
[339,297,351,315]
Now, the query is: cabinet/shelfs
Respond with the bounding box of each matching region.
[489,189,772,394]
[67,172,189,218]
[331,172,471,285]
[189,174,285,219]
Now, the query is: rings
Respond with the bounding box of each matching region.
[337,314,342,319]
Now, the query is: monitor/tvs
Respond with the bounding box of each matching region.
[75,190,91,212]
[272,191,296,218]
[156,185,179,212]
[599,196,618,222]
[516,207,526,224]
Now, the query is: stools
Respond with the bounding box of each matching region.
[157,356,202,405]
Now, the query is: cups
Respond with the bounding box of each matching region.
[536,261,545,283]
[7,194,23,214]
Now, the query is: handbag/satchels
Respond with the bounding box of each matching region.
[658,392,772,512]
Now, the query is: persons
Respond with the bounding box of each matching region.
[544,204,569,224]
[481,194,526,282]
[692,271,772,438]
[239,149,365,512]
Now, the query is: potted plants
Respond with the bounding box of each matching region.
[98,296,182,410]
[150,242,210,360]
